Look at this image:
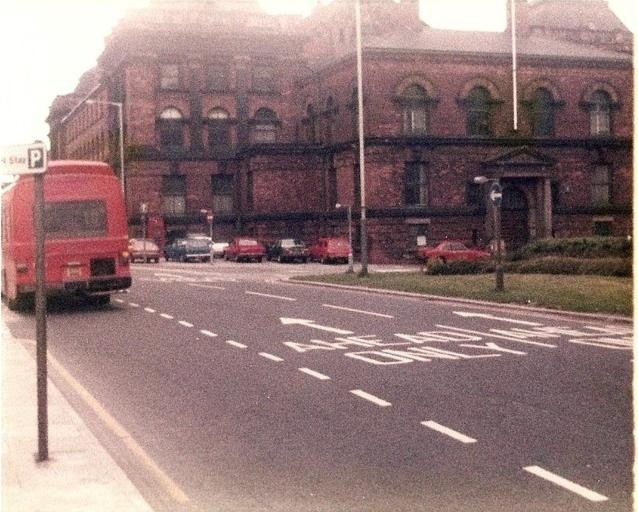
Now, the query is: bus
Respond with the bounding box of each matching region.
[1,159,131,310]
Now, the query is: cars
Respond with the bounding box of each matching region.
[130,235,352,263]
[414,240,494,267]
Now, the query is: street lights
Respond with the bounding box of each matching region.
[472,174,506,292]
[199,208,214,262]
[335,203,353,274]
[79,95,124,218]
